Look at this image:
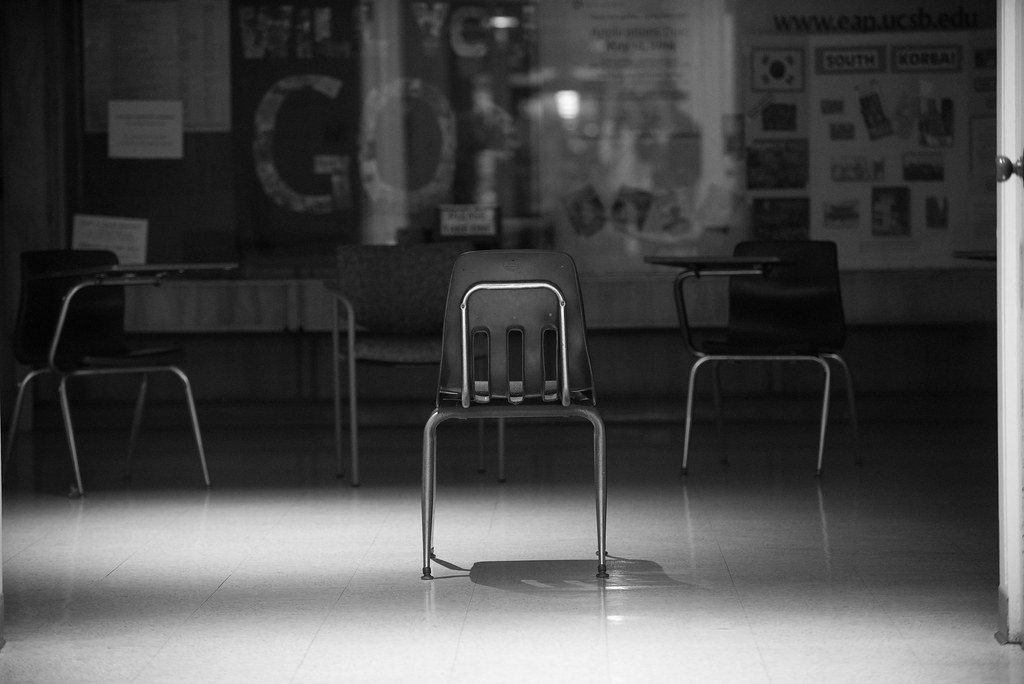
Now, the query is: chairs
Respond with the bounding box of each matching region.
[327,243,506,482]
[422,250,608,579]
[643,240,864,479]
[0,248,213,497]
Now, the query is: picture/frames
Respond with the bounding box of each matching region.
[750,47,806,93]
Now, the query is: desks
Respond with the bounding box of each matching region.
[48,262,244,371]
[642,257,799,347]
